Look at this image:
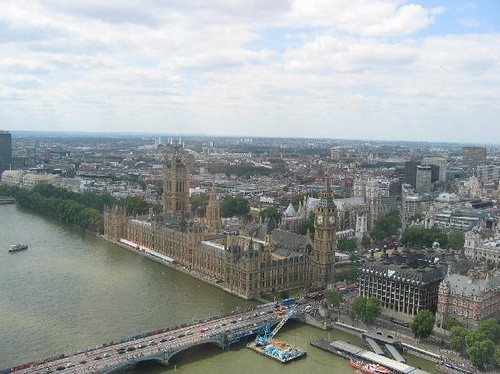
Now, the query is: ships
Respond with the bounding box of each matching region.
[350,357,391,374]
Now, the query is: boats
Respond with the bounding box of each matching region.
[8,243,28,252]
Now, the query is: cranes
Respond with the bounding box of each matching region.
[255,302,300,346]
